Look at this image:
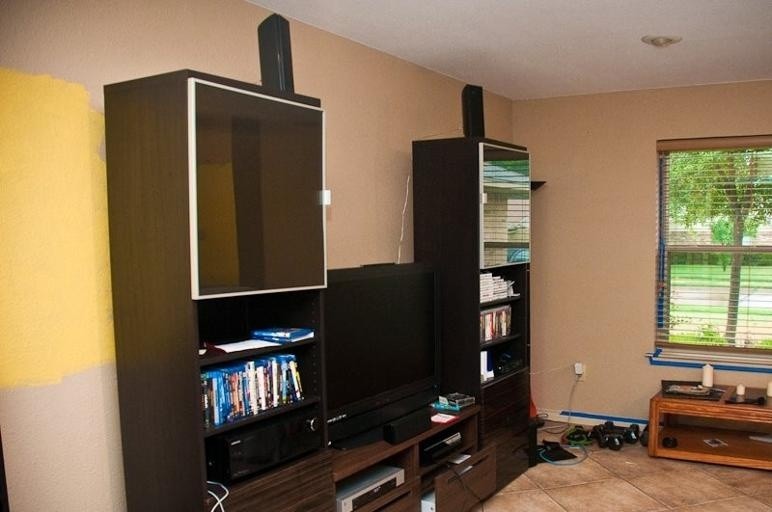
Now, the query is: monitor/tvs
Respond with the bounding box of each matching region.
[326,261,444,451]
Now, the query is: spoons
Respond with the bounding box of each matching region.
[692,385,725,393]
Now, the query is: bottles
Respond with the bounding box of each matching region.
[703,362,714,388]
[767,380,772,397]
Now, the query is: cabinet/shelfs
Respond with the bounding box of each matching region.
[332,403,497,512]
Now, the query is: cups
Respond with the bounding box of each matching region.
[736,383,745,395]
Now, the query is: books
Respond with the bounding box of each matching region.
[480,350,488,382]
[480,272,515,342]
[200,327,314,428]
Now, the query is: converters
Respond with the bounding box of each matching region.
[575,363,583,375]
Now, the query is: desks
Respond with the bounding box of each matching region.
[647,388,772,471]
